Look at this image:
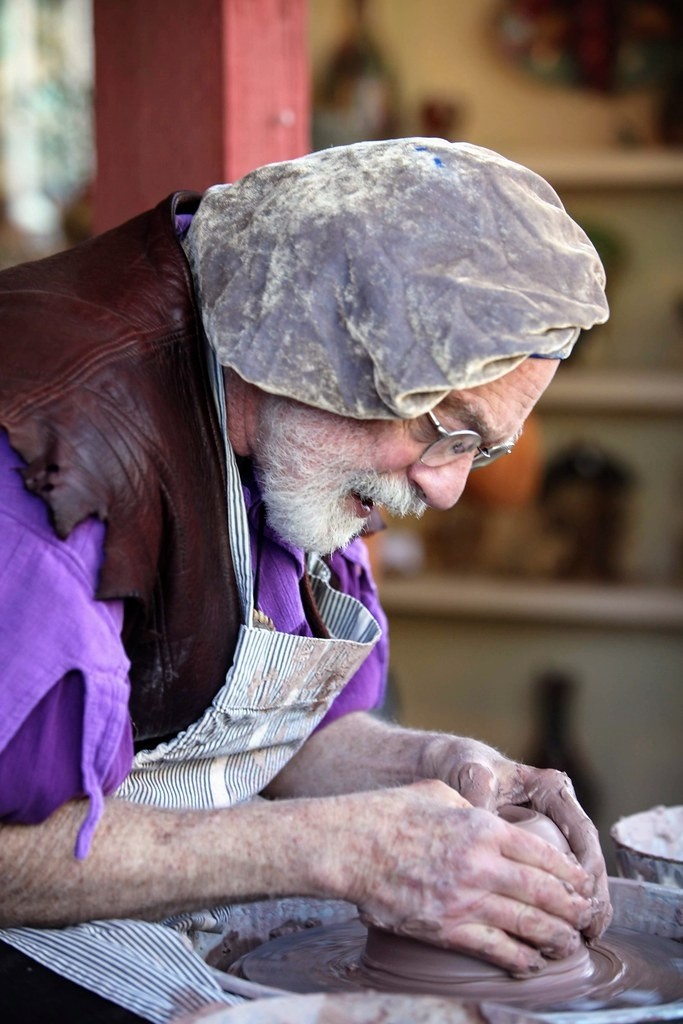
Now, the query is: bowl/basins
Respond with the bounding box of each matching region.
[609,805,683,889]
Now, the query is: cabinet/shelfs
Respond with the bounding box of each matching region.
[378,146,683,631]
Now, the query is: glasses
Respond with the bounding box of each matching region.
[418,410,515,470]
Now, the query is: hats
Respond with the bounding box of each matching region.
[184,137,610,422]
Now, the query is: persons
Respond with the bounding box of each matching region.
[0,134,613,1023]
